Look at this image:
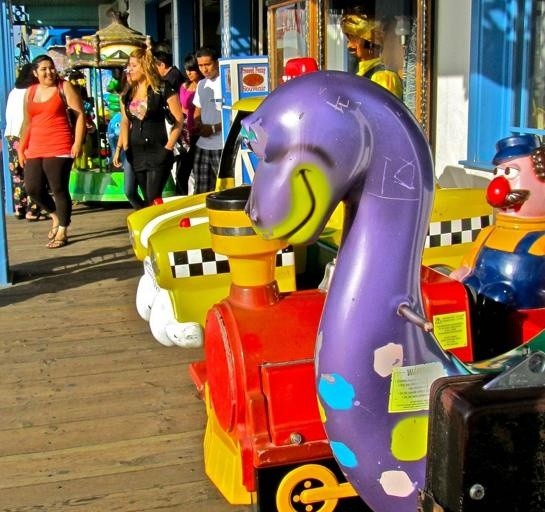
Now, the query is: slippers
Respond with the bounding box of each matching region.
[47,226,67,248]
[16,211,46,220]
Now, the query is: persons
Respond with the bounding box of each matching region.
[119,48,184,204]
[3,63,52,222]
[112,63,149,210]
[18,55,87,249]
[154,51,196,196]
[340,13,403,104]
[175,54,204,196]
[191,47,222,193]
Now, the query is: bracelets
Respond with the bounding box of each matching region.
[115,146,122,150]
[212,124,216,135]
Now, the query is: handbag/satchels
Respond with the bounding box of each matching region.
[161,103,175,124]
[66,109,88,143]
